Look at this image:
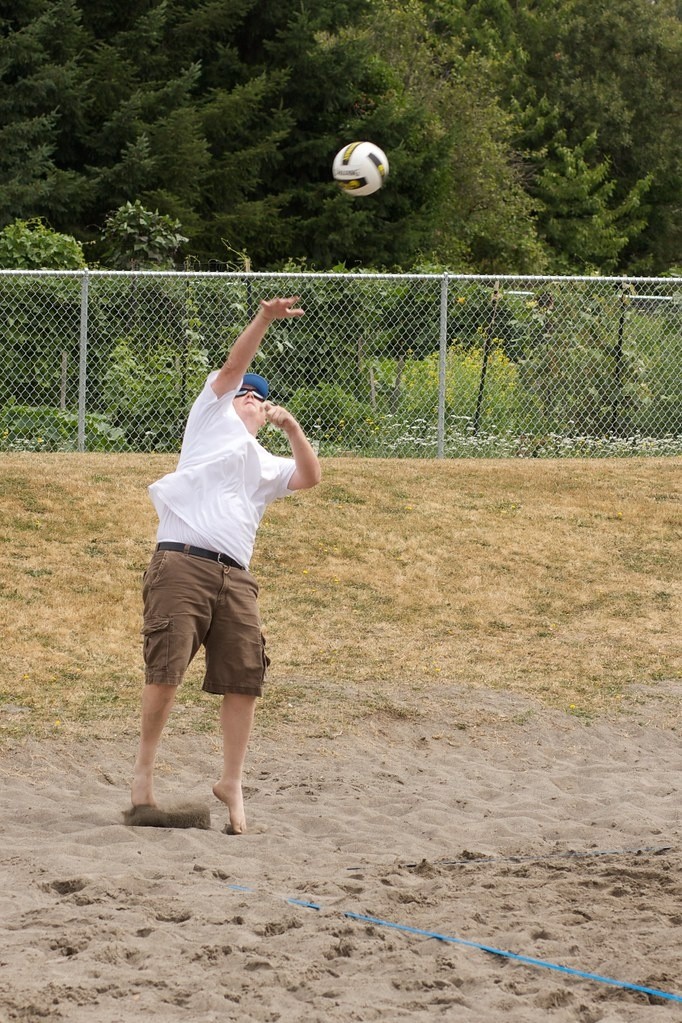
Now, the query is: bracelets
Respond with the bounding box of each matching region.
[261,313,270,320]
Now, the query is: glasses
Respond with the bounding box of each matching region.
[234,387,266,402]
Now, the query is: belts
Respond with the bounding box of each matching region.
[159,542,246,569]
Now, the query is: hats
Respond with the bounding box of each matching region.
[243,373,270,399]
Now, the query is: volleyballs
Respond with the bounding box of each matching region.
[331,141,390,198]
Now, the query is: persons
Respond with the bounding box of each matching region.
[130,297,320,835]
